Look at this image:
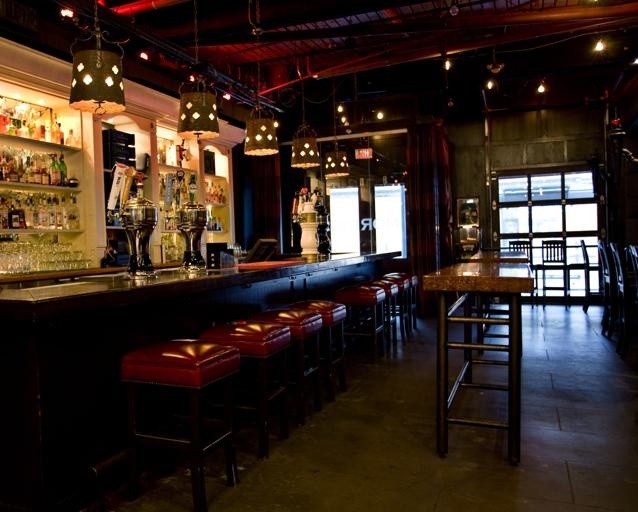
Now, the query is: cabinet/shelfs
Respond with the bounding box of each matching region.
[205,172,227,234]
[157,161,200,268]
[0,131,86,236]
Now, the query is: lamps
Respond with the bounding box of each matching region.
[176,0,221,143]
[322,82,350,178]
[291,81,321,169]
[242,46,279,157]
[68,1,130,117]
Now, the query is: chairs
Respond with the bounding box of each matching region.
[580,239,637,368]
[509,240,539,310]
[541,239,572,311]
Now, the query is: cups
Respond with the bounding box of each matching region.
[0,241,92,274]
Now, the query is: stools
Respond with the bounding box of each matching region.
[336,284,383,361]
[118,338,243,512]
[362,279,398,346]
[378,275,408,343]
[384,273,419,335]
[201,318,294,461]
[273,299,347,403]
[242,308,324,426]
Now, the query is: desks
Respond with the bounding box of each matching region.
[468,248,529,356]
[419,262,535,466]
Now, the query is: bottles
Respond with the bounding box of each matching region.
[207,215,224,231]
[0,146,68,187]
[205,182,226,204]
[160,207,180,229]
[0,106,77,148]
[0,191,80,231]
[159,170,197,200]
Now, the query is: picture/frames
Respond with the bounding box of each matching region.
[455,195,481,229]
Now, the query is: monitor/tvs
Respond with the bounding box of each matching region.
[244,239,278,263]
